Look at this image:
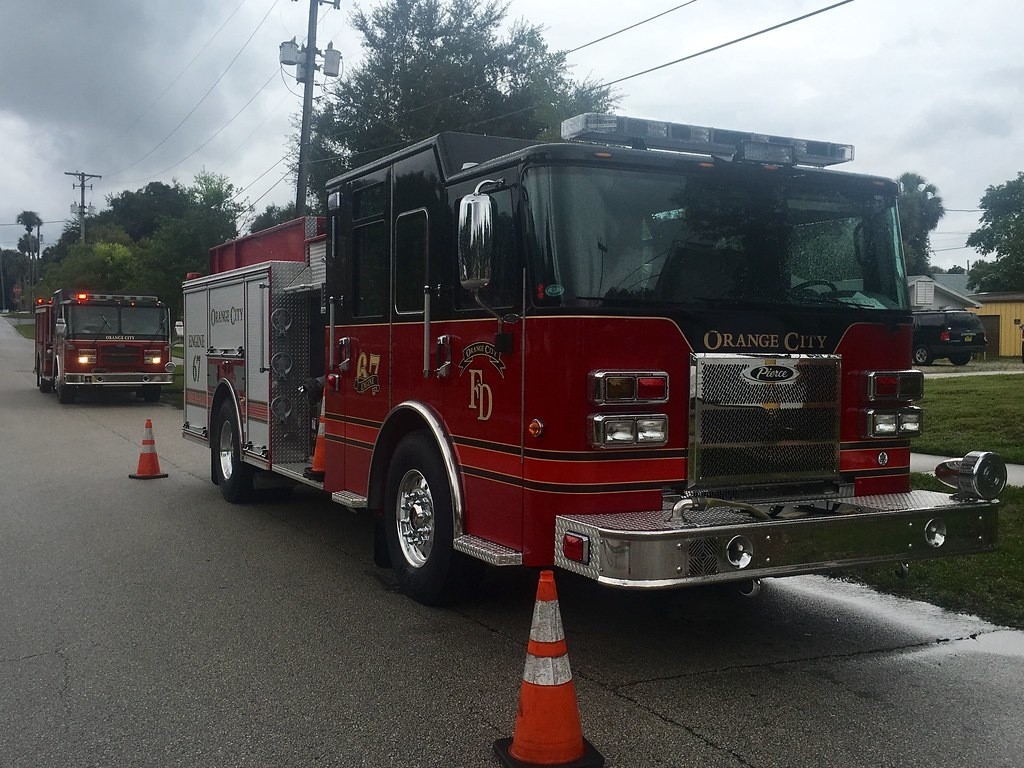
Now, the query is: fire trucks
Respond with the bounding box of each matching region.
[33,290,176,403]
[181,109,1008,606]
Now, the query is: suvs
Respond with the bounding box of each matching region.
[912,308,988,366]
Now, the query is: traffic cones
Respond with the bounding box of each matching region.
[493,569,606,768]
[128,419,169,479]
[303,385,325,480]
